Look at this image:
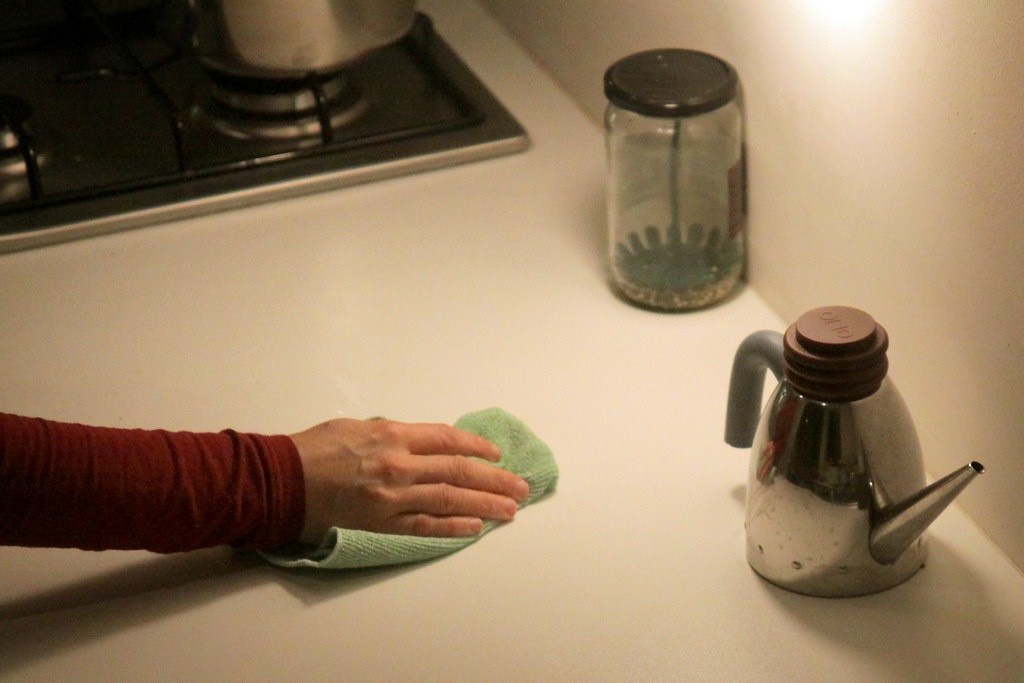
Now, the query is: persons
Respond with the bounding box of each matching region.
[0,411,529,554]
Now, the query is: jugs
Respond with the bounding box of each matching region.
[724,305,986,599]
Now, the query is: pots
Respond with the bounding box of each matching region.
[190,0,417,76]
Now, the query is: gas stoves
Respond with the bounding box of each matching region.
[0,0,533,256]
